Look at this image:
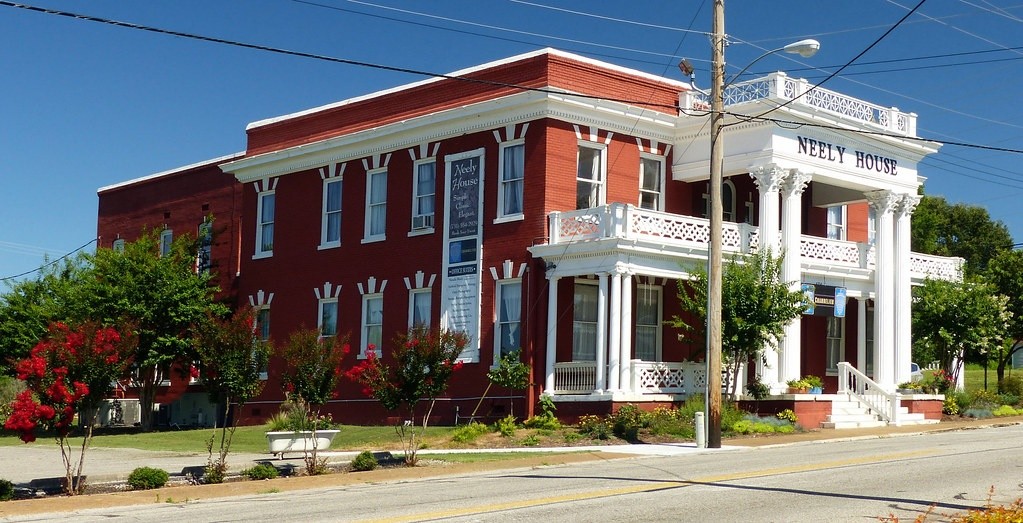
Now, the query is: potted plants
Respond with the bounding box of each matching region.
[898,382,929,394]
[786,380,799,394]
[797,381,810,393]
[804,376,823,394]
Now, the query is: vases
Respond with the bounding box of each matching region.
[266,430,341,453]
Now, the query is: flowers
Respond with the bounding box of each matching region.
[265,399,333,432]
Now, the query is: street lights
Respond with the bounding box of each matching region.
[679,37,819,449]
